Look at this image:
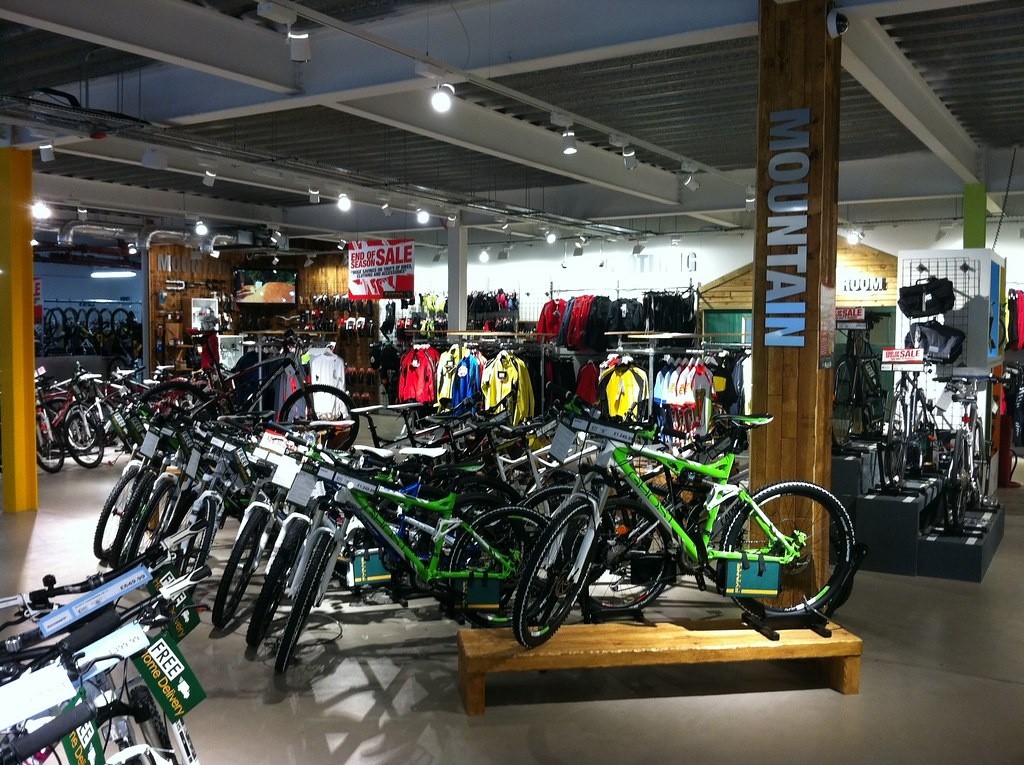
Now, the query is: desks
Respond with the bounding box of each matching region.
[33,356,130,382]
[453,618,865,714]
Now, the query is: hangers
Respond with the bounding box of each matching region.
[424,286,680,302]
[548,349,634,368]
[246,341,335,357]
[662,341,752,366]
[411,338,528,358]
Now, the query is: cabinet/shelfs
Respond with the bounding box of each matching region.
[891,248,1007,495]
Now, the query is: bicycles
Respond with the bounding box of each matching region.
[832,307,1013,535]
[35,312,360,473]
[93,392,858,671]
[0,519,211,765]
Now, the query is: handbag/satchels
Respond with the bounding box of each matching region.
[898,281,954,316]
[906,320,966,363]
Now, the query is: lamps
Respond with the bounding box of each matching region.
[26,129,139,254]
[608,134,638,170]
[267,224,282,243]
[196,158,220,188]
[256,1,312,63]
[745,186,755,213]
[185,212,210,237]
[338,238,347,250]
[550,112,578,154]
[308,187,320,204]
[670,235,682,247]
[849,228,859,244]
[375,191,619,270]
[337,183,351,211]
[633,236,648,255]
[200,244,220,259]
[304,255,317,268]
[681,162,701,191]
[936,222,955,243]
[268,253,279,265]
[414,61,456,112]
[859,225,874,241]
